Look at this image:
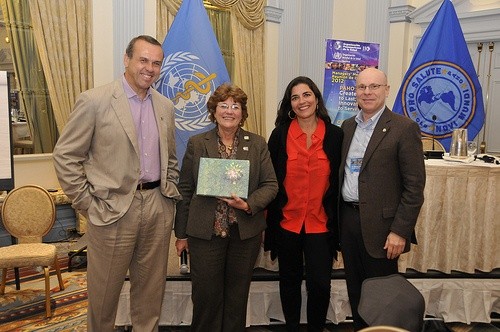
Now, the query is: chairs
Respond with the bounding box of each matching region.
[0,184,64,318]
[421,136,446,154]
[14,140,34,150]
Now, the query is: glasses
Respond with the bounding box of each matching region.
[357,84,387,90]
[217,103,240,111]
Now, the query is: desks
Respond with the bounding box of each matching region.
[123,154,500,280]
[12,122,31,154]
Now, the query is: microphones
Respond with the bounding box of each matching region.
[180,249,189,274]
[432,115,437,150]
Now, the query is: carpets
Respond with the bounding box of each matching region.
[0,257,126,332]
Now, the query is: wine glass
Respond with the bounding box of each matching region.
[467,141,477,157]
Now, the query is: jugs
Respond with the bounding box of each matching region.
[449,129,468,159]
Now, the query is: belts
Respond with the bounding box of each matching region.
[136,179,160,190]
[343,201,360,209]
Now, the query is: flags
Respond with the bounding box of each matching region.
[392,0,486,155]
[150,0,231,171]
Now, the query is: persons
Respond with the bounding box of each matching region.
[337,68,426,332]
[264,76,343,332]
[174,85,278,332]
[328,62,378,72]
[52,35,183,332]
